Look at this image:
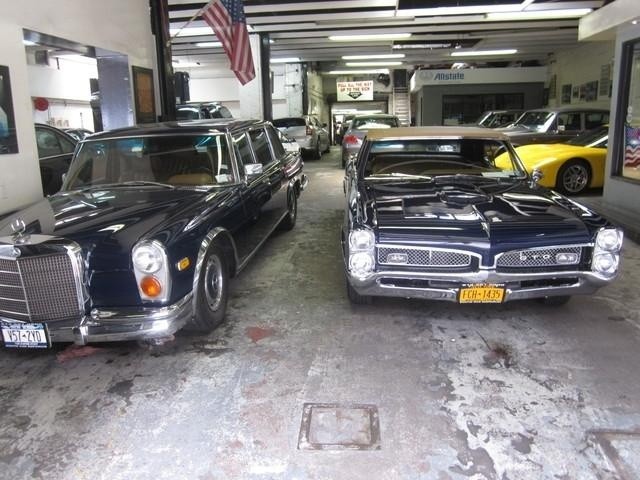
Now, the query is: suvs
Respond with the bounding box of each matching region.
[474,106,609,136]
[173,99,232,120]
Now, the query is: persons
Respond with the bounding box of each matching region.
[335,120,344,146]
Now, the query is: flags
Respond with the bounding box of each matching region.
[201,0,256,86]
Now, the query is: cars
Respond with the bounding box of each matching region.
[272,115,332,159]
[336,114,401,168]
